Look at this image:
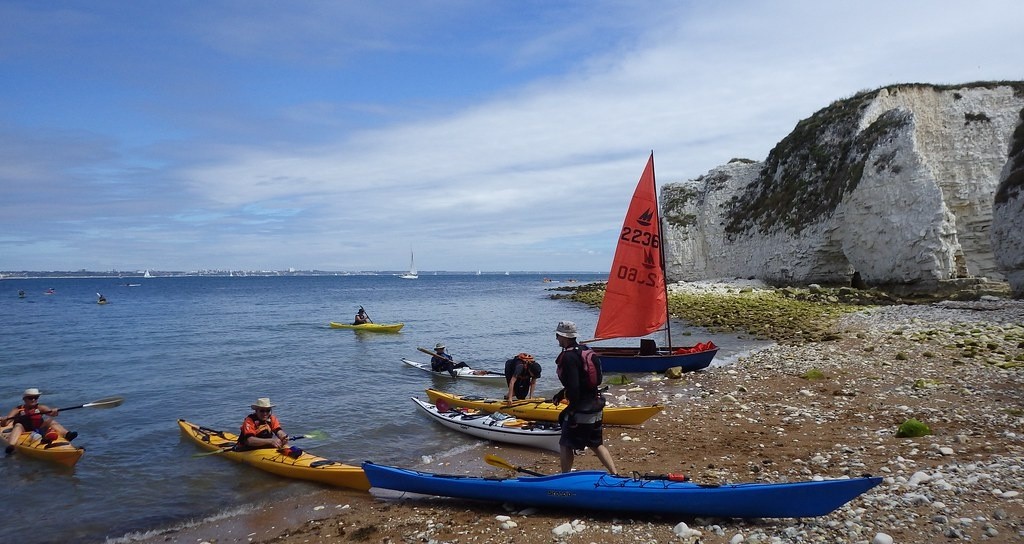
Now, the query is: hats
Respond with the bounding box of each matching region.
[358,309,365,314]
[21,388,42,399]
[554,320,581,338]
[251,398,276,410]
[529,362,542,378]
[433,343,446,351]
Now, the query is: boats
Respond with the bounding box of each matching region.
[18,290,26,298]
[406,394,564,455]
[1,417,92,469]
[328,319,405,335]
[45,291,54,295]
[426,386,663,434]
[360,457,884,523]
[401,356,513,384]
[175,418,374,494]
[97,299,107,305]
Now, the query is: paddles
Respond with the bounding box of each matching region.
[417,347,458,364]
[360,305,373,324]
[484,454,547,477]
[5,397,124,421]
[192,434,312,457]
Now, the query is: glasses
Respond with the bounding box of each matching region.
[256,409,271,413]
[24,396,39,400]
[555,333,561,339]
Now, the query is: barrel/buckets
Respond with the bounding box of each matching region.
[641,339,656,356]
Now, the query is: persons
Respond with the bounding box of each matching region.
[0,388,78,455]
[503,353,542,406]
[430,342,472,379]
[232,397,291,453]
[353,308,369,324]
[553,320,617,474]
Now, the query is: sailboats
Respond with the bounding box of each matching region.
[397,250,420,279]
[580,153,721,376]
